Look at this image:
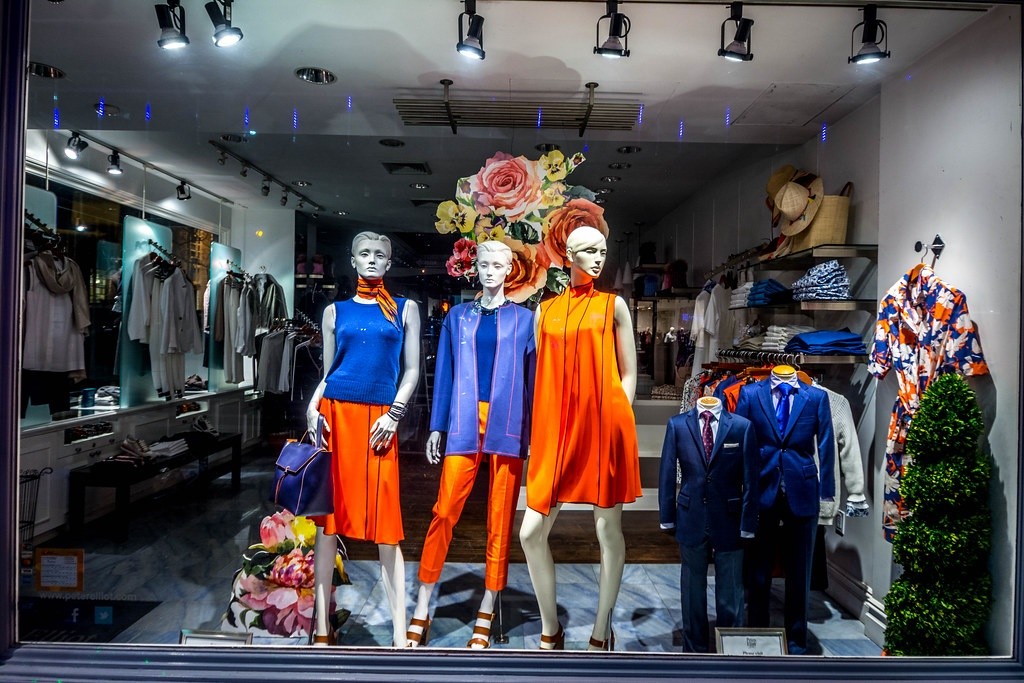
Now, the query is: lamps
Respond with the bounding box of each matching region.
[176,181,191,201]
[717,0,754,62]
[593,0,631,59]
[311,207,319,219]
[218,152,228,166]
[848,3,891,64]
[279,187,289,206]
[64,132,89,159]
[204,0,243,47]
[155,0,190,49]
[297,197,304,209]
[106,150,123,175]
[456,0,486,61]
[261,177,272,197]
[240,162,248,177]
[613,222,647,290]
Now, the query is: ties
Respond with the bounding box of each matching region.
[701,410,713,462]
[774,382,793,438]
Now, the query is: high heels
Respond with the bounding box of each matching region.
[313,622,338,647]
[467,611,495,649]
[406,612,431,647]
[540,620,565,650]
[587,626,615,651]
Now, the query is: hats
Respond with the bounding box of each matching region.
[764,163,824,237]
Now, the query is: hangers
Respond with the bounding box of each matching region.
[146,240,182,276]
[225,259,267,290]
[275,310,323,346]
[702,255,746,293]
[23,209,65,261]
[909,243,928,281]
[715,348,812,386]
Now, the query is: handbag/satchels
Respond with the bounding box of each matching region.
[270,412,333,518]
[791,180,854,251]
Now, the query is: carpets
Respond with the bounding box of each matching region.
[19,594,163,643]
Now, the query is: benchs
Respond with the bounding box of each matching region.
[66,430,243,550]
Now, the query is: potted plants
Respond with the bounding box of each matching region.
[882,374,995,657]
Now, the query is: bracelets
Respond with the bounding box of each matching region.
[387,401,407,421]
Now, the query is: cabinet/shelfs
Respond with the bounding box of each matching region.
[18,384,267,549]
[714,243,878,365]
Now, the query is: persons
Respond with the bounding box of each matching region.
[734,364,835,652]
[658,397,760,654]
[306,232,423,648]
[519,227,643,654]
[406,240,536,649]
